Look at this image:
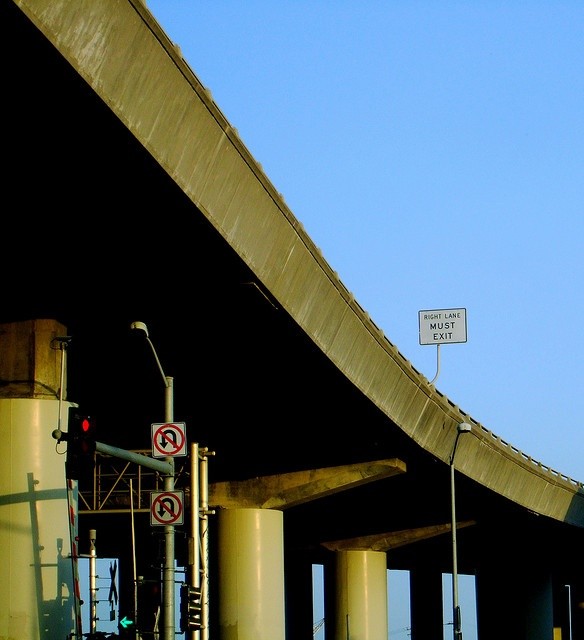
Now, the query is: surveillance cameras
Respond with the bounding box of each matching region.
[55,334,75,343]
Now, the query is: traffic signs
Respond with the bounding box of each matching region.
[418,307,469,345]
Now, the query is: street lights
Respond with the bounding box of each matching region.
[449,422,474,640]
[130,319,176,640]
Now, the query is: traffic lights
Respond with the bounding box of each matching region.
[118,615,135,629]
[181,583,203,630]
[66,405,97,482]
